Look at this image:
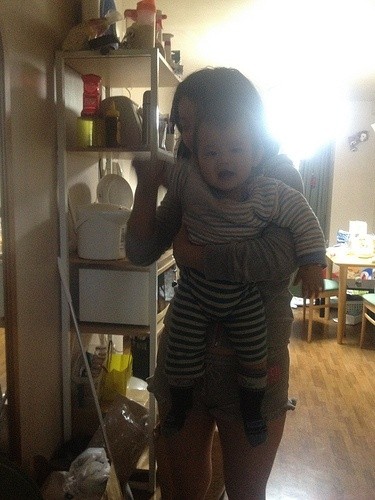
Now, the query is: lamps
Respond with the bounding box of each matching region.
[349,129,368,151]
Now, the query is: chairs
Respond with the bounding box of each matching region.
[360,293,375,348]
[303,279,339,342]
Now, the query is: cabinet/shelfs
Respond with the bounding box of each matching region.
[61,47,183,494]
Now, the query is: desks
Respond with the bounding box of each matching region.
[325,242,375,343]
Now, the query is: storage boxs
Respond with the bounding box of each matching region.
[328,298,367,325]
[40,392,150,500]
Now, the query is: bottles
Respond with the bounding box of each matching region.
[134,0,172,67]
[75,73,121,147]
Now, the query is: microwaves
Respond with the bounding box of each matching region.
[79,253,180,328]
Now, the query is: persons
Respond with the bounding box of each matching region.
[125,66,327,500]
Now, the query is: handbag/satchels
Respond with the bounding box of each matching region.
[99,340,133,407]
